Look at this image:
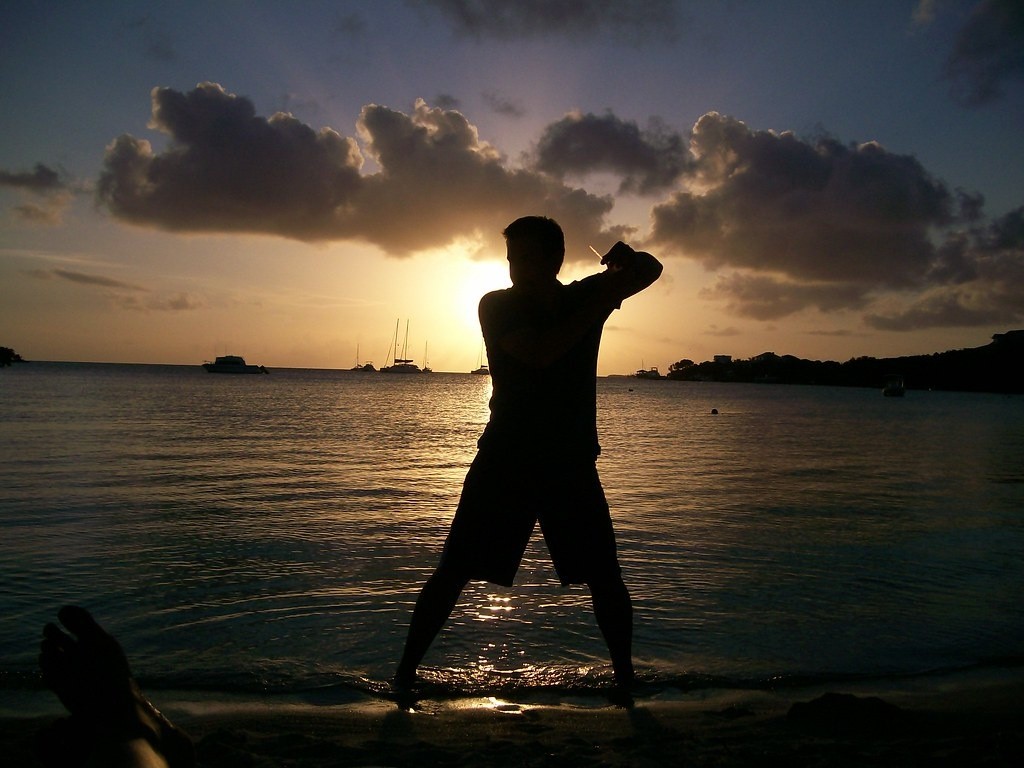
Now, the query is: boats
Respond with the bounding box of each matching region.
[882,374,906,397]
[636,359,660,379]
[202,355,270,375]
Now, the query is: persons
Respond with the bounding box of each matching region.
[389,215,664,708]
[38,605,172,768]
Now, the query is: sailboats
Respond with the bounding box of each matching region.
[471,337,489,375]
[351,344,377,372]
[423,342,432,373]
[379,318,424,373]
